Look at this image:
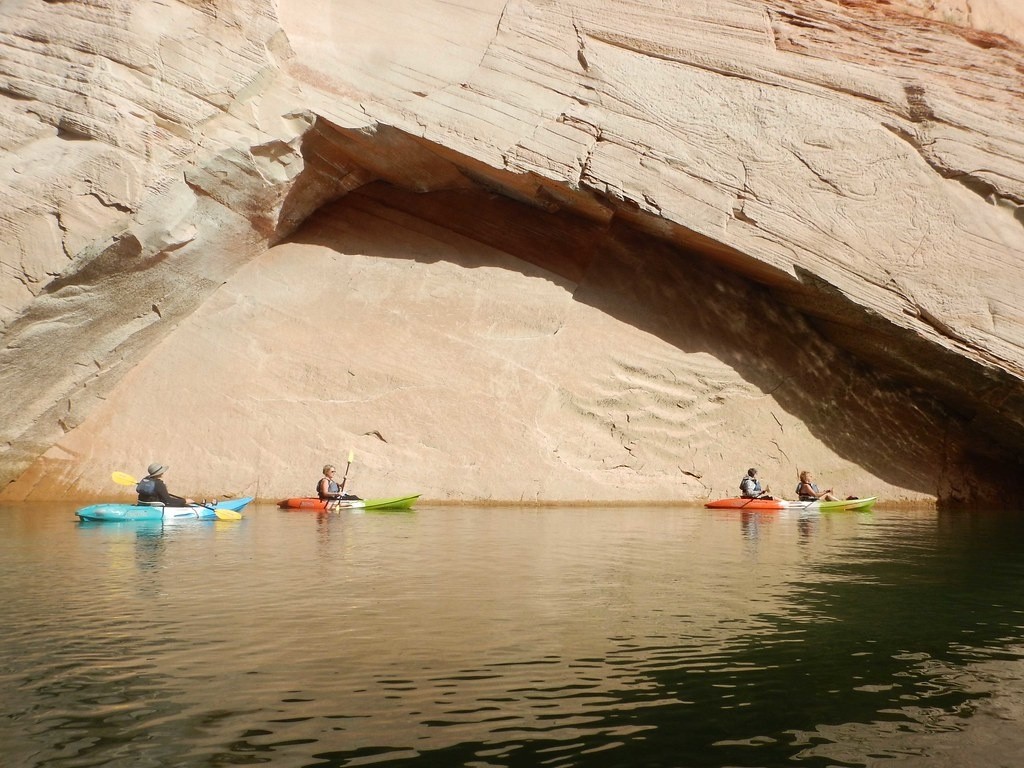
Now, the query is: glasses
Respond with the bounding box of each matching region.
[332,471,336,473]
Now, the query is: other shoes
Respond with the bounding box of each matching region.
[210,499,218,506]
[200,499,206,507]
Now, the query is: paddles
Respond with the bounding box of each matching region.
[803,489,833,509]
[111,472,241,520]
[761,485,772,498]
[333,450,354,512]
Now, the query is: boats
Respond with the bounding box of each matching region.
[703,495,882,511]
[277,492,427,509]
[73,494,254,524]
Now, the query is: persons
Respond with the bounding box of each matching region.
[137,462,218,508]
[795,471,859,501]
[316,465,363,500]
[739,468,785,501]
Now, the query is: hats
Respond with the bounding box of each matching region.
[148,463,169,477]
